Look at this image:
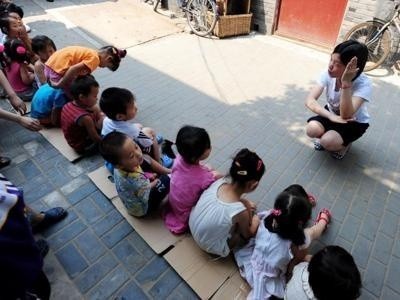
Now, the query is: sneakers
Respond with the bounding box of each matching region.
[1,156,10,168]
[335,144,351,159]
[312,139,323,151]
[32,207,67,230]
[318,208,331,229]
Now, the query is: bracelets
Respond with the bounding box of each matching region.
[342,82,353,89]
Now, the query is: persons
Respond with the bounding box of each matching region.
[188,147,265,260]
[162,125,220,235]
[304,40,371,160]
[0,1,175,220]
[0,156,68,299]
[283,245,364,300]
[233,184,333,300]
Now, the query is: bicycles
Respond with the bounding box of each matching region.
[344,0,400,75]
[152,0,219,37]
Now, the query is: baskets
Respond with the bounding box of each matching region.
[207,10,252,38]
